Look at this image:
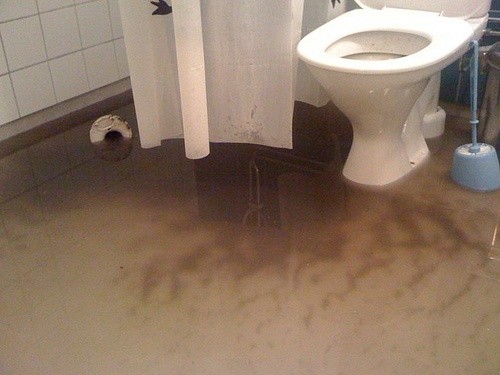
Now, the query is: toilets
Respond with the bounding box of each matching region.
[297,0,491,186]
